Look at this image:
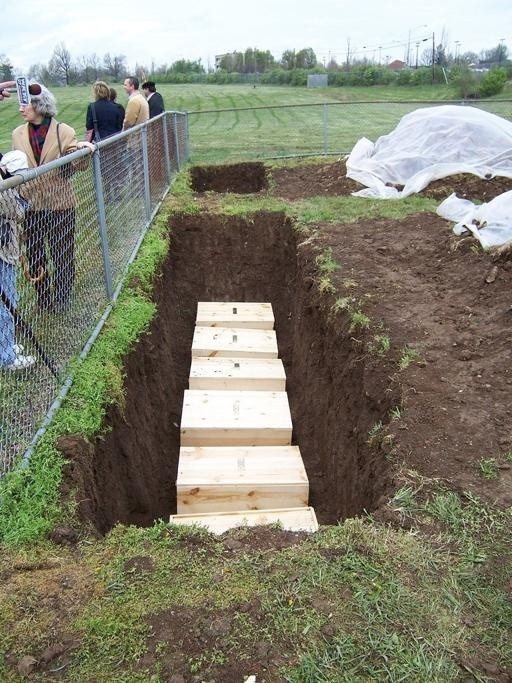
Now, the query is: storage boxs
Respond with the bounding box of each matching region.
[166,300,320,534]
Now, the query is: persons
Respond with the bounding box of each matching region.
[86,81,124,204]
[8,78,96,313]
[0,148,39,374]
[109,87,126,117]
[0,79,17,102]
[122,75,148,196]
[143,81,164,116]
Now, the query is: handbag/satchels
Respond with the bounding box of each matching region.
[85,129,101,144]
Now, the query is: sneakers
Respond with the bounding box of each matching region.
[0,344,37,370]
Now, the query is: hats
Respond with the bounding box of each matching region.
[141,82,155,89]
